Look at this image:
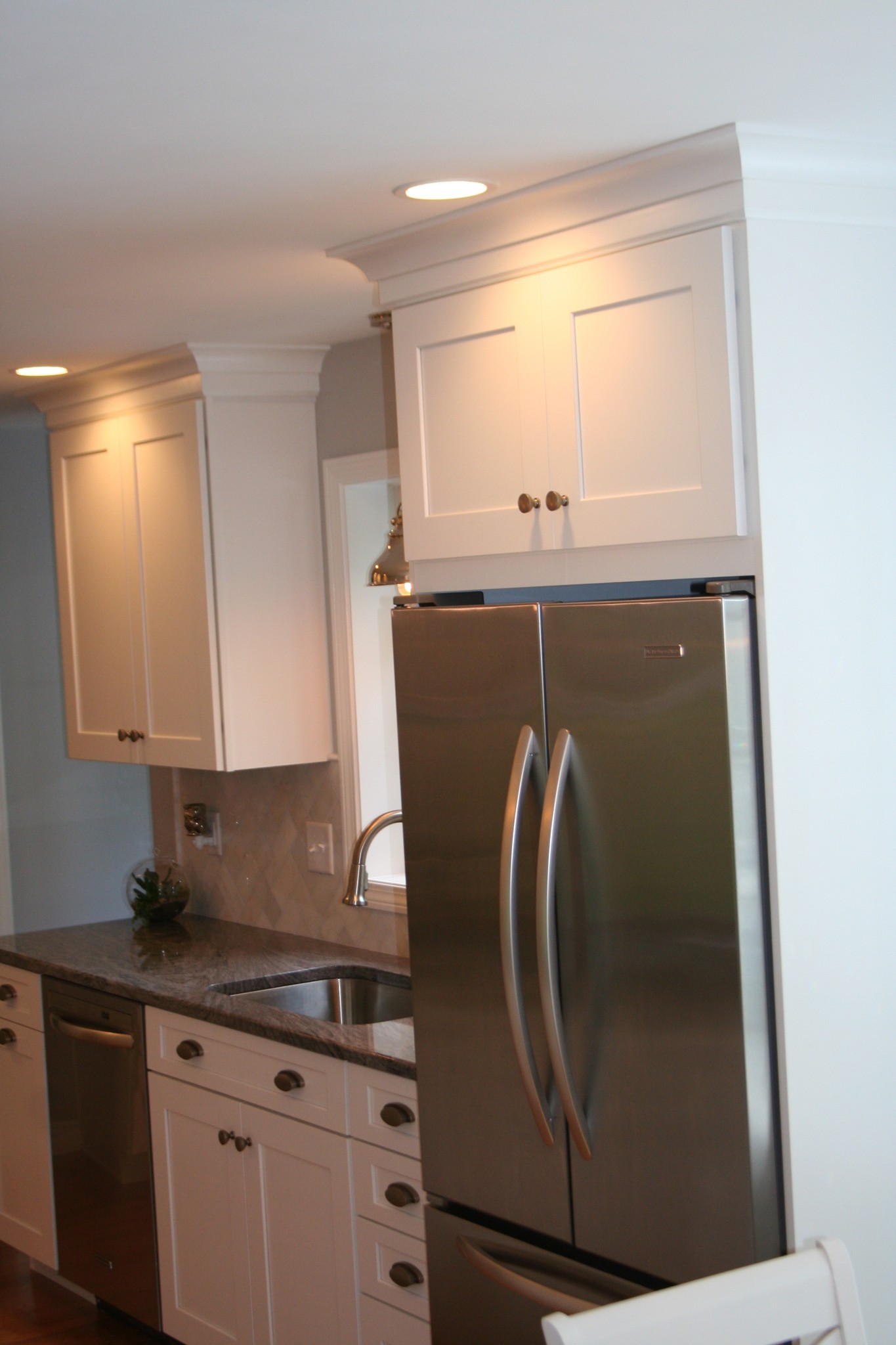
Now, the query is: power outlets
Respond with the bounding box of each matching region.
[200,811,223,856]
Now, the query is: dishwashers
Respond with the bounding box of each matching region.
[42,975,161,1330]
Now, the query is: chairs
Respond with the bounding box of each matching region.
[538,1236,868,1345]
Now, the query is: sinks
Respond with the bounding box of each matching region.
[208,962,414,1026]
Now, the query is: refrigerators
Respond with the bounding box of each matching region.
[390,592,788,1344]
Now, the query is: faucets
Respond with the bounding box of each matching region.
[342,808,404,907]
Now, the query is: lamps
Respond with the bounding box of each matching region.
[364,500,411,597]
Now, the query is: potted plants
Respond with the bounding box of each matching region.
[120,855,193,935]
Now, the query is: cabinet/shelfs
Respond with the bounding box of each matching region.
[0,962,59,1272]
[324,122,764,598]
[145,1003,432,1345]
[11,341,334,775]
